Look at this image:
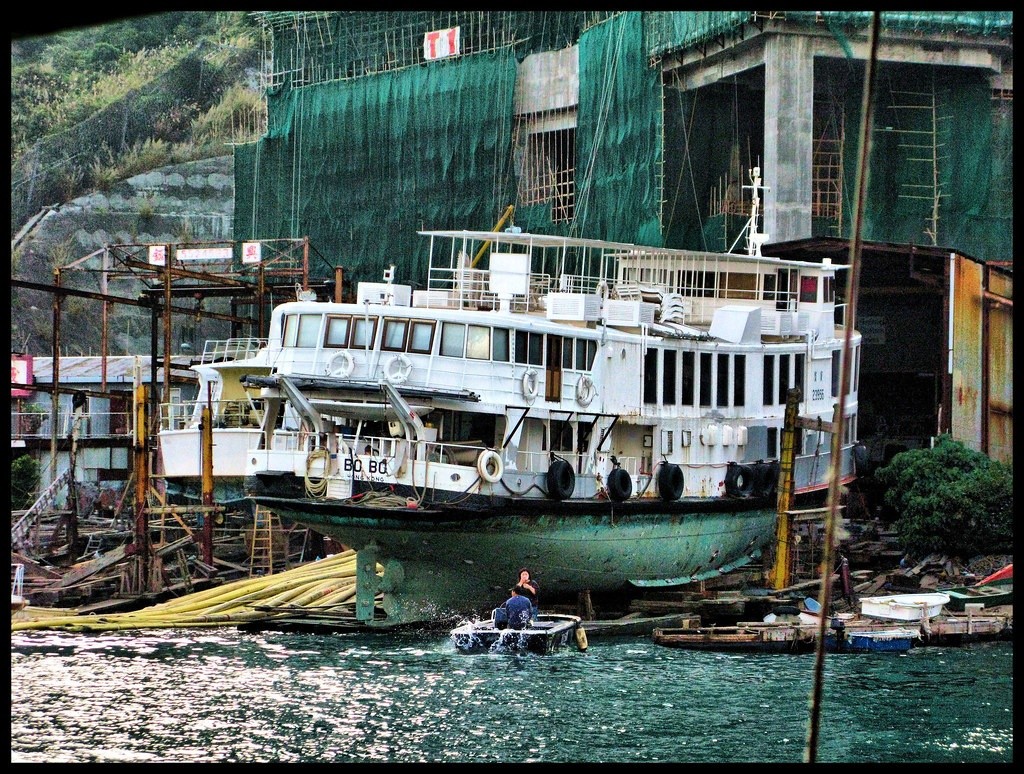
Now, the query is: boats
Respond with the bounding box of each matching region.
[150,167,1019,659]
[450,603,589,657]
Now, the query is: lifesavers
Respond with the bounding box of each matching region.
[325,350,355,378]
[548,461,575,499]
[523,368,539,399]
[607,468,633,500]
[754,463,780,496]
[595,280,609,308]
[726,465,754,497]
[476,450,504,482]
[383,354,412,384]
[577,377,594,406]
[659,464,684,499]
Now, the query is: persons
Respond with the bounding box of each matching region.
[365,444,374,456]
[506,568,541,630]
